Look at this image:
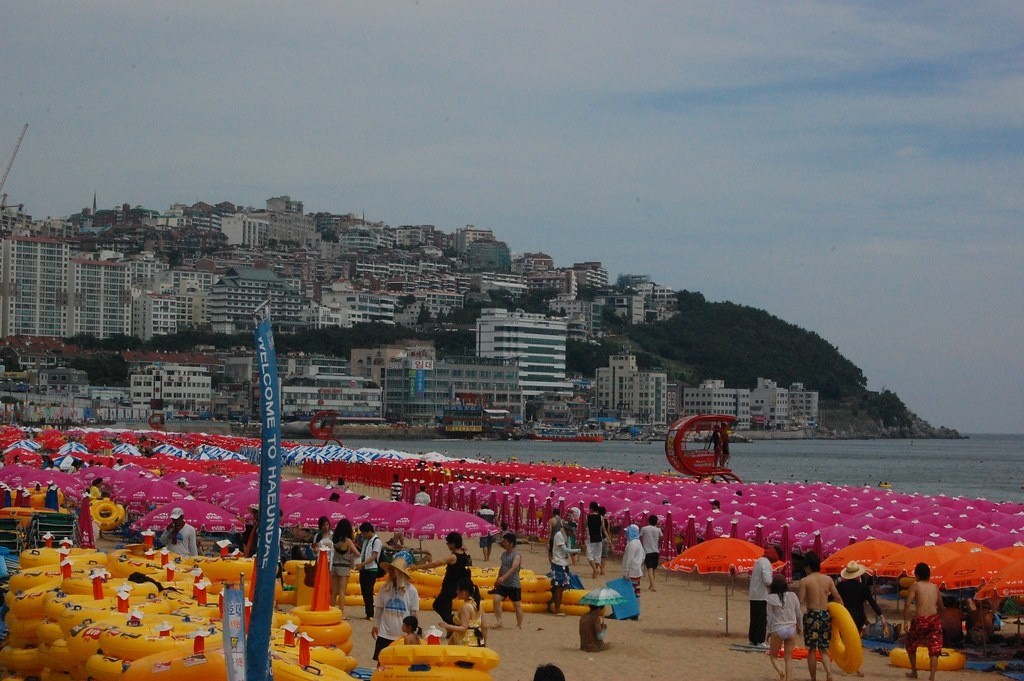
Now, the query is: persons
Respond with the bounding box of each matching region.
[14,430,527,644]
[712,421,729,469]
[533,467,1003,681]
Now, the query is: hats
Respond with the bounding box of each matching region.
[170,507,184,519]
[248,503,259,513]
[623,524,640,543]
[388,557,412,580]
[840,560,866,579]
[178,477,189,485]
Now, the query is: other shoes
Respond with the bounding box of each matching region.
[756,642,771,650]
[748,642,755,647]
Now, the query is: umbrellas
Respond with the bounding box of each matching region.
[0,424,500,556]
[42,526,445,670]
[489,462,1024,646]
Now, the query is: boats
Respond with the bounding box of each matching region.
[551,434,604,442]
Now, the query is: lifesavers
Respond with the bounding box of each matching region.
[899,577,917,598]
[151,470,160,475]
[9,486,65,508]
[827,600,864,673]
[429,466,451,480]
[0,506,69,546]
[90,497,126,531]
[0,545,609,681]
[889,645,966,671]
